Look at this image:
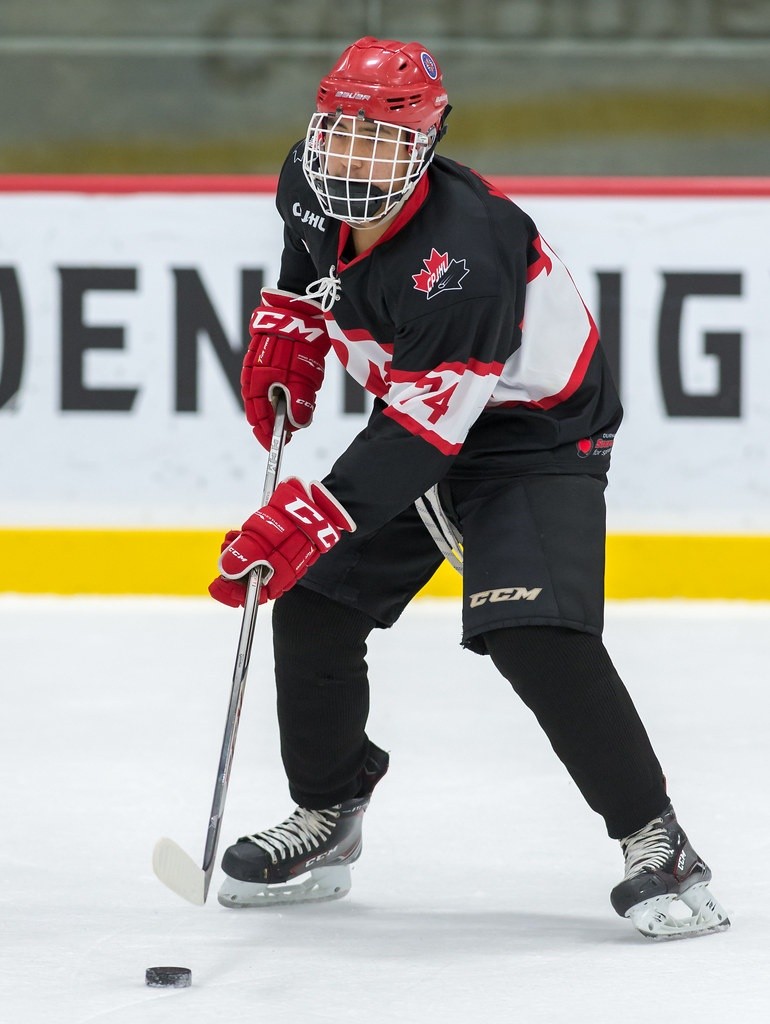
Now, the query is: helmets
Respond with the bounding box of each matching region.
[302,37,449,225]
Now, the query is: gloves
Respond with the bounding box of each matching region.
[207,476,356,608]
[240,287,332,452]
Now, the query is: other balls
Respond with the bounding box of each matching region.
[145,966,193,989]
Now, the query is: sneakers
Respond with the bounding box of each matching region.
[217,742,389,909]
[611,803,731,939]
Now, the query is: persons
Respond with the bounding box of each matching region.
[209,37,731,939]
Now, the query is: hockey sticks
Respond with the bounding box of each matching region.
[148,391,291,908]
